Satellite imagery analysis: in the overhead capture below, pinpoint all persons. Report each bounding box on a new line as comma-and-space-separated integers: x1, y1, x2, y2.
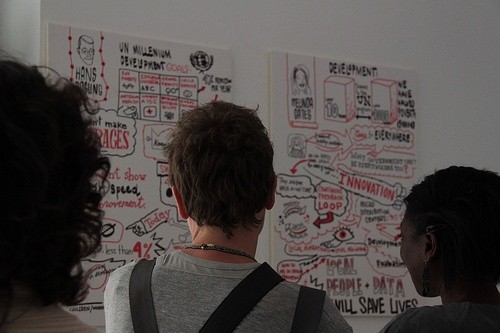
0, 47, 112, 333
375, 165, 500, 333
102, 96, 356, 333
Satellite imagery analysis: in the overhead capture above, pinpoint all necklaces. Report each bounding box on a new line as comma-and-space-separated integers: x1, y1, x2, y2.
183, 243, 258, 264
